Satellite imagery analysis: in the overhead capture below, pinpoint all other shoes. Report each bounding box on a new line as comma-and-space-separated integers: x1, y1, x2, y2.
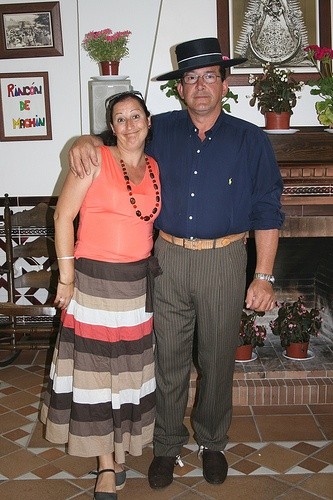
114, 463, 127, 490
202, 446, 229, 484
148, 454, 177, 489
88, 469, 118, 500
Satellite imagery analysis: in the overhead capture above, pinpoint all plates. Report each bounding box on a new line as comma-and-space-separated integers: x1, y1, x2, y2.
90, 76, 130, 81
263, 128, 301, 134
282, 349, 315, 360
235, 351, 258, 363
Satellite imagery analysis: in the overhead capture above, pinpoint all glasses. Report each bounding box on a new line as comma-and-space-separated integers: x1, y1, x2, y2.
105, 90, 146, 113
182, 73, 222, 84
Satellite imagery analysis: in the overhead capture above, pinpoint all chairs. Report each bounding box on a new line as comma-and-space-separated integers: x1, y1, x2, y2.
0, 194, 79, 368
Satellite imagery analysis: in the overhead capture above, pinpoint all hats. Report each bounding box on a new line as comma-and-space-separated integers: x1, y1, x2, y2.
155, 37, 249, 81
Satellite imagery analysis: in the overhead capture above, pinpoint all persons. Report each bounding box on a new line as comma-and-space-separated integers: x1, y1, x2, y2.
38, 91, 161, 500
68, 38, 284, 489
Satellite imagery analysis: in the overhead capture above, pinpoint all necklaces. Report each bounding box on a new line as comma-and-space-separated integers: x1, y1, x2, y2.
118, 146, 160, 222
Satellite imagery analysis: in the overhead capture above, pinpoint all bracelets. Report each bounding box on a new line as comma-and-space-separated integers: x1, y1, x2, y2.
255, 272, 275, 286
57, 256, 76, 260
58, 279, 75, 286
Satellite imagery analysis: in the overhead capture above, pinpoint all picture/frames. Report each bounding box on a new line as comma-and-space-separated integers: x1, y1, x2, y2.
0, 2, 63, 60
215, 0, 333, 87
0, 72, 52, 142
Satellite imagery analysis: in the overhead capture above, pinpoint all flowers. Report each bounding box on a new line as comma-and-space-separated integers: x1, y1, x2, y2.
246, 63, 304, 114
270, 296, 324, 347
302, 45, 333, 128
82, 29, 131, 62
238, 311, 268, 348
223, 56, 230, 61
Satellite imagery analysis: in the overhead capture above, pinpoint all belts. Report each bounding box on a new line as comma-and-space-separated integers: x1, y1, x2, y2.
158, 229, 250, 251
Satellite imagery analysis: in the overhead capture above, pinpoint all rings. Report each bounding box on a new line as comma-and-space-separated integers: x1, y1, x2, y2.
60, 298, 64, 302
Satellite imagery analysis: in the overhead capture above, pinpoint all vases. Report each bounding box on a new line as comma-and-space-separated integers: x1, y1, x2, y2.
98, 61, 121, 76
263, 111, 292, 130
236, 336, 253, 361
286, 335, 311, 359
